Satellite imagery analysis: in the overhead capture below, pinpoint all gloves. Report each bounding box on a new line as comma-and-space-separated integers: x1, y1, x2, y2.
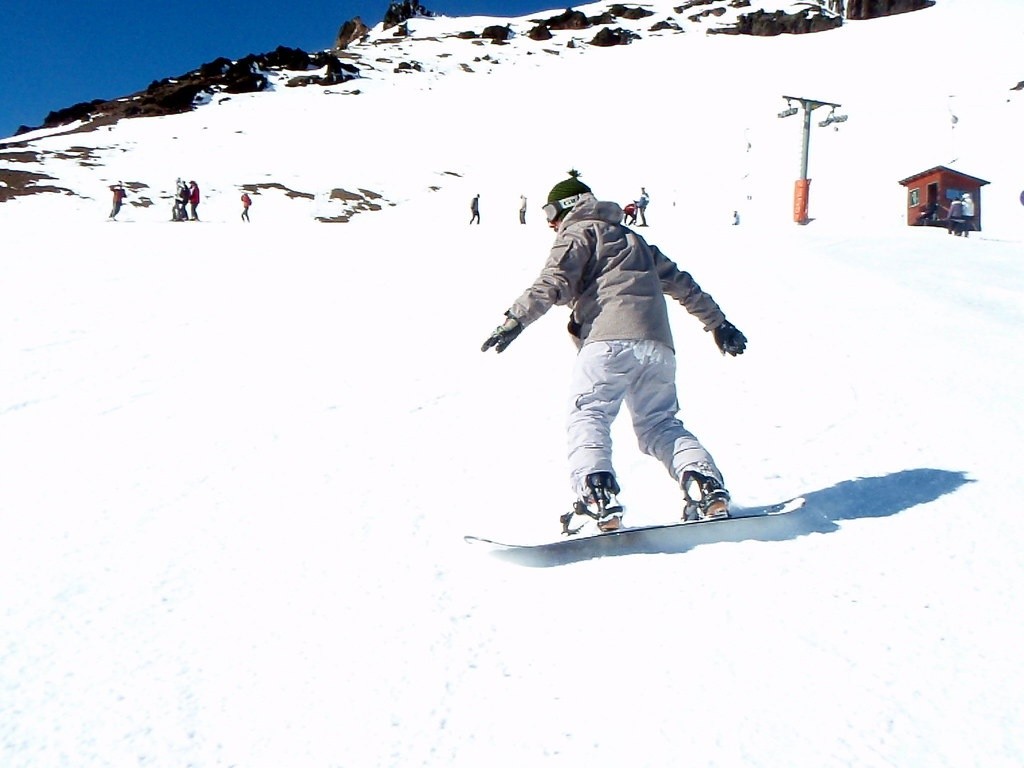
710, 319, 748, 357
481, 314, 523, 354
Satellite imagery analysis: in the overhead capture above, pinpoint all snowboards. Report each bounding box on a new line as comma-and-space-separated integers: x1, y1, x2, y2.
461, 495, 808, 551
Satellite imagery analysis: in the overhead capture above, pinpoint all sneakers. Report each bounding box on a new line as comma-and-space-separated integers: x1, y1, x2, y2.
560, 494, 625, 535
682, 477, 729, 521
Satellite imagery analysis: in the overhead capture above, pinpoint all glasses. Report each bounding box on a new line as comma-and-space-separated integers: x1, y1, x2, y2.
542, 192, 594, 222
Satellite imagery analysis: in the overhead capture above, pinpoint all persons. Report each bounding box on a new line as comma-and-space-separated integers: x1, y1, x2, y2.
947, 196, 963, 236
732, 211, 740, 225
180, 181, 191, 221
187, 181, 200, 221
634, 187, 649, 226
109, 180, 126, 221
623, 204, 639, 225
480, 178, 748, 536
519, 195, 528, 224
469, 194, 480, 225
958, 193, 974, 236
241, 193, 252, 222
171, 179, 183, 222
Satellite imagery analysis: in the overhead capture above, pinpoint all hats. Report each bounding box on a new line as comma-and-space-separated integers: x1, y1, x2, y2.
547, 169, 597, 222
